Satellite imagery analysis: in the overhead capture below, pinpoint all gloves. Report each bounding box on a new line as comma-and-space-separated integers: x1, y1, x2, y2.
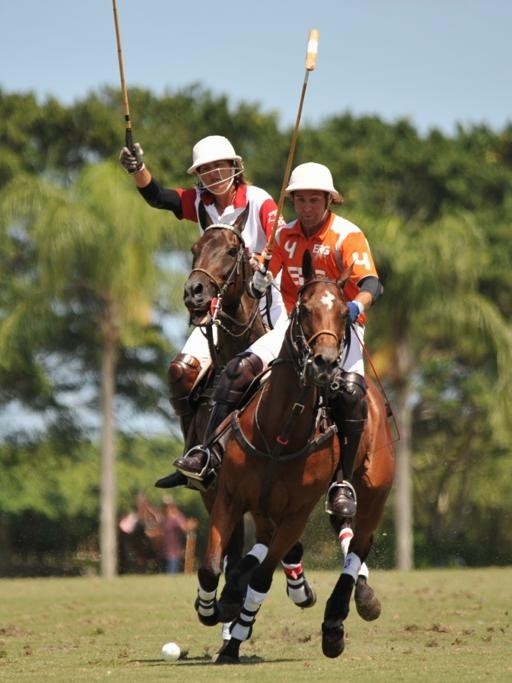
347, 302, 359, 323
253, 270, 273, 293
119, 143, 145, 174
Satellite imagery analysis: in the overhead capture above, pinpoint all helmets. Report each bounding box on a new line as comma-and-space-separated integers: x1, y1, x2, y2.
285, 162, 338, 199
186, 135, 241, 174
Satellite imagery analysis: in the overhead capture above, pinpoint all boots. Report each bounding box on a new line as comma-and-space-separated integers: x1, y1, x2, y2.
330, 480, 357, 518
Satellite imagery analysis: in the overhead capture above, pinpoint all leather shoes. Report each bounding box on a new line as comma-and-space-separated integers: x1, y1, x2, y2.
155, 452, 218, 491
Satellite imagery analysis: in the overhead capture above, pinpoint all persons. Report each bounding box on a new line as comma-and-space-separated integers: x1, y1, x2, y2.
116, 134, 288, 488
173, 162, 388, 518
156, 493, 198, 574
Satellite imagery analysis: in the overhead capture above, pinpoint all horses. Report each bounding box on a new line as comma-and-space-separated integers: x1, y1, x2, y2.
181, 197, 318, 665
193, 247, 400, 667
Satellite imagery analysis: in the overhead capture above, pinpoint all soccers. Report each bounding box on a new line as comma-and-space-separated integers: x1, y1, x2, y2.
162, 643, 181, 663
